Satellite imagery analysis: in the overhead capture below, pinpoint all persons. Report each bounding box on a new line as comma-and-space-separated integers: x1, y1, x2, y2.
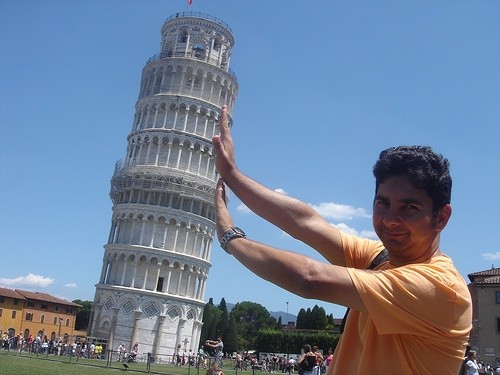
214, 105, 473, 375
0, 325, 333, 375
459, 342, 500, 375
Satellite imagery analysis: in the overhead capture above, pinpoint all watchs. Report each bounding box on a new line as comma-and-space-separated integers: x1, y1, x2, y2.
219, 226, 247, 256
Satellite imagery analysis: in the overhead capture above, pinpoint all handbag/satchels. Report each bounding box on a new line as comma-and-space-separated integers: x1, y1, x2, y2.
300, 355, 313, 372
